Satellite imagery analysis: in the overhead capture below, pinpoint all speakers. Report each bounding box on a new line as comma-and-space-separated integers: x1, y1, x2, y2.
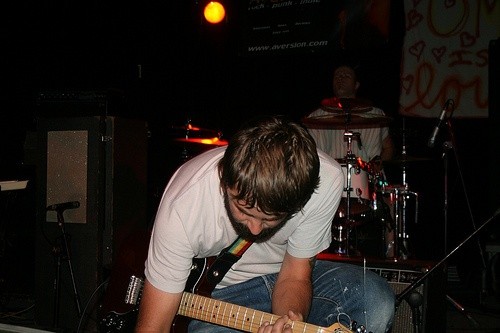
363, 263, 447, 333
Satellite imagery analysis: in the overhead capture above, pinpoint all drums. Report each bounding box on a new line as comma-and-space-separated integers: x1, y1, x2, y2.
334, 158, 422, 263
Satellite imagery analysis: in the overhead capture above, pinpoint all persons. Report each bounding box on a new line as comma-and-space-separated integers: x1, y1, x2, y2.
133, 119, 396, 333
303, 61, 396, 259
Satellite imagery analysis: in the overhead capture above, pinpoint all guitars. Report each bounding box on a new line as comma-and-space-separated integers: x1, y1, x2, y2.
75, 256, 373, 333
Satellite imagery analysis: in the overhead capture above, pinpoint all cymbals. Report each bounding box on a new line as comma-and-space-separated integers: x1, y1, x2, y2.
320, 95, 375, 114
301, 114, 393, 131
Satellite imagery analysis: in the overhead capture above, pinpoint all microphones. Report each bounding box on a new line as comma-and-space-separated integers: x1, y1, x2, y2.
431, 99, 449, 143
48, 201, 80, 211
